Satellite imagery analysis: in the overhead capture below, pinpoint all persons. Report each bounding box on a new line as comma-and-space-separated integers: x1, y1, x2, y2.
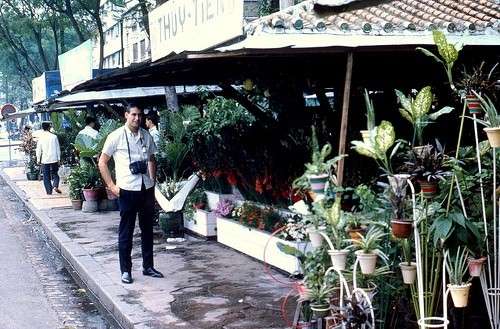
145, 114, 160, 151
75, 116, 98, 163
98, 102, 163, 284
36, 122, 64, 194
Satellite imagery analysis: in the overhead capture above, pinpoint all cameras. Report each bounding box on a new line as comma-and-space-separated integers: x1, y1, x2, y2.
129, 160, 147, 175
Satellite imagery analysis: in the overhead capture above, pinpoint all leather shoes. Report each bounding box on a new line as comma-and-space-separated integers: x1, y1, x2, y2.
143, 267, 163, 277
121, 272, 132, 283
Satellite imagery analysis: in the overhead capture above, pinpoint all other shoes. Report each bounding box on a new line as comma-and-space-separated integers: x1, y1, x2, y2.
54, 187, 62, 193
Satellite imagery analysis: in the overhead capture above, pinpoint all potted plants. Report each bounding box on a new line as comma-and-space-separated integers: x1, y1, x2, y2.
26, 30, 500, 329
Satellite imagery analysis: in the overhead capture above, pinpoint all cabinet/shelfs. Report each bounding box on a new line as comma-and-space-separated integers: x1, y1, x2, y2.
215, 200, 324, 276
182, 190, 231, 236
